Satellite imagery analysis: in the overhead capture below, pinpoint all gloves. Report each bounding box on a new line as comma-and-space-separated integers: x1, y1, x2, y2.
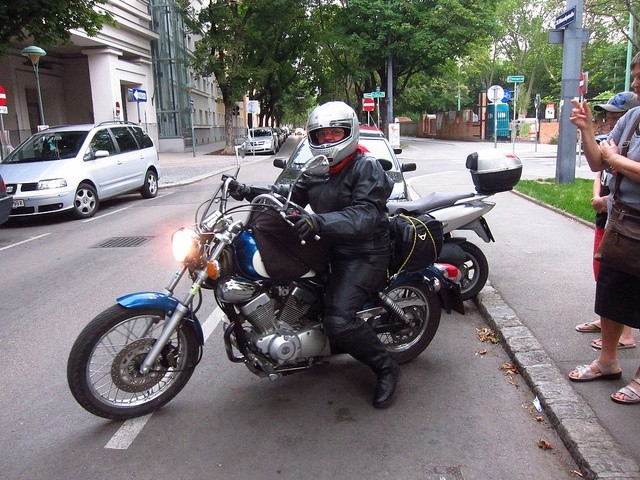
228, 184, 251, 201
285, 206, 321, 237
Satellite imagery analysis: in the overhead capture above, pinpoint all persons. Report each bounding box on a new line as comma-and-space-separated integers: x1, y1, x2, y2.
508, 119, 513, 141
228, 101, 400, 409
569, 54, 640, 405
514, 120, 521, 141
575, 91, 639, 350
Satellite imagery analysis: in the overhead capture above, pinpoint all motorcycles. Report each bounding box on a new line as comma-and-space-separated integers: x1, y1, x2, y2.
67, 136, 467, 422
380, 150, 523, 307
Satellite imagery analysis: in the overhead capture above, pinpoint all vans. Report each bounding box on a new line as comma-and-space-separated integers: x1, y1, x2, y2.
3, 121, 162, 222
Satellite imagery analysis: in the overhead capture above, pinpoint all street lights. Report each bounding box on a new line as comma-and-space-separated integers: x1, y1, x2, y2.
20, 45, 48, 126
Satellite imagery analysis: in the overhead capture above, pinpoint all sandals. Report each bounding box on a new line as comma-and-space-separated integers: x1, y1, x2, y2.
610, 377, 640, 404
568, 359, 623, 382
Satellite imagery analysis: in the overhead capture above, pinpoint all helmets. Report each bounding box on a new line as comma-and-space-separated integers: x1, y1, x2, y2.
306, 101, 360, 167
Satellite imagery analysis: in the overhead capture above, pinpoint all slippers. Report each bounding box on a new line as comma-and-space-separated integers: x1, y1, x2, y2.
575, 322, 601, 332
591, 339, 637, 350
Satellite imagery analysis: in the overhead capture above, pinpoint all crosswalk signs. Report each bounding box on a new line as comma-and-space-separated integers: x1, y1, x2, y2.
501, 89, 512, 103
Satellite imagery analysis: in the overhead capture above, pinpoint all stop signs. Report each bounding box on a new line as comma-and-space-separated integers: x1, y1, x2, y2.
113, 101, 120, 116
578, 73, 589, 100
362, 98, 375, 112
0, 85, 8, 115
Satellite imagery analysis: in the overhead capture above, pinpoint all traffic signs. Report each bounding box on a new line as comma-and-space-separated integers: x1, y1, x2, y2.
128, 88, 147, 102
363, 91, 385, 98
247, 100, 259, 114
507, 76, 525, 83
534, 95, 542, 111
555, 5, 576, 30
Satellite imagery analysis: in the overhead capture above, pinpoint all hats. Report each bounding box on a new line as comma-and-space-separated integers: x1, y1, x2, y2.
593, 91, 639, 113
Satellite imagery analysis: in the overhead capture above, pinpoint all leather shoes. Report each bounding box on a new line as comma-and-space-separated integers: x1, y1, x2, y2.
371, 359, 400, 408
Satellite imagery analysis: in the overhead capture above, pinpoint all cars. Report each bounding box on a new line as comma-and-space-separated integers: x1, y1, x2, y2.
274, 126, 293, 143
244, 127, 279, 155
295, 128, 307, 136
271, 135, 418, 203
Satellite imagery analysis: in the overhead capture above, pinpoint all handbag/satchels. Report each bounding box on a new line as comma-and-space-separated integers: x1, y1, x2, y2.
389, 208, 443, 272
594, 114, 640, 279
